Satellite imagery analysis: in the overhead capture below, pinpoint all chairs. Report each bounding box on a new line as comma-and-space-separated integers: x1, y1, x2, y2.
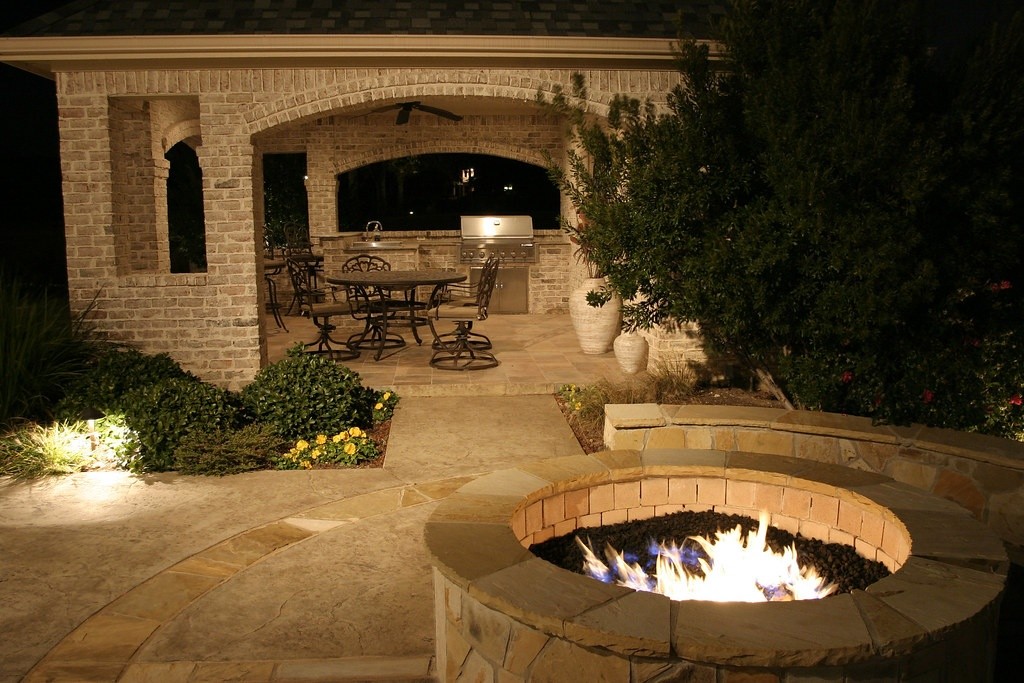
255, 221, 500, 371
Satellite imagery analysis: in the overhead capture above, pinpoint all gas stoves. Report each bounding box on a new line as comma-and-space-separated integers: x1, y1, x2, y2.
458, 216, 534, 263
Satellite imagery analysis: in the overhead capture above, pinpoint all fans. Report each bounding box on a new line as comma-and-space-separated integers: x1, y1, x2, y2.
372, 100, 462, 126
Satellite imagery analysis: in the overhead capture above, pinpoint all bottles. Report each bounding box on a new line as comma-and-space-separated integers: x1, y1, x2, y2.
373, 224, 380, 241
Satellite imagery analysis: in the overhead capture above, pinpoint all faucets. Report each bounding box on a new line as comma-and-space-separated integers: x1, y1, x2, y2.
366, 221, 382, 241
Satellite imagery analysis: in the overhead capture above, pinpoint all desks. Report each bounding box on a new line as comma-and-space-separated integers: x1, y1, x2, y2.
324, 271, 467, 363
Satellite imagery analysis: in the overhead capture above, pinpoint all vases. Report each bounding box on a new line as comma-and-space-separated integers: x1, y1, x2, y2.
614, 327, 648, 376
568, 277, 624, 354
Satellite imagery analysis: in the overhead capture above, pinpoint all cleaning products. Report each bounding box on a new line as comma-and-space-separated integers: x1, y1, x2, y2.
372, 224, 381, 241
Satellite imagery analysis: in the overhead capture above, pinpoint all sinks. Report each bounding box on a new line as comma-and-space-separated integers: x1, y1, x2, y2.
352, 242, 403, 247
